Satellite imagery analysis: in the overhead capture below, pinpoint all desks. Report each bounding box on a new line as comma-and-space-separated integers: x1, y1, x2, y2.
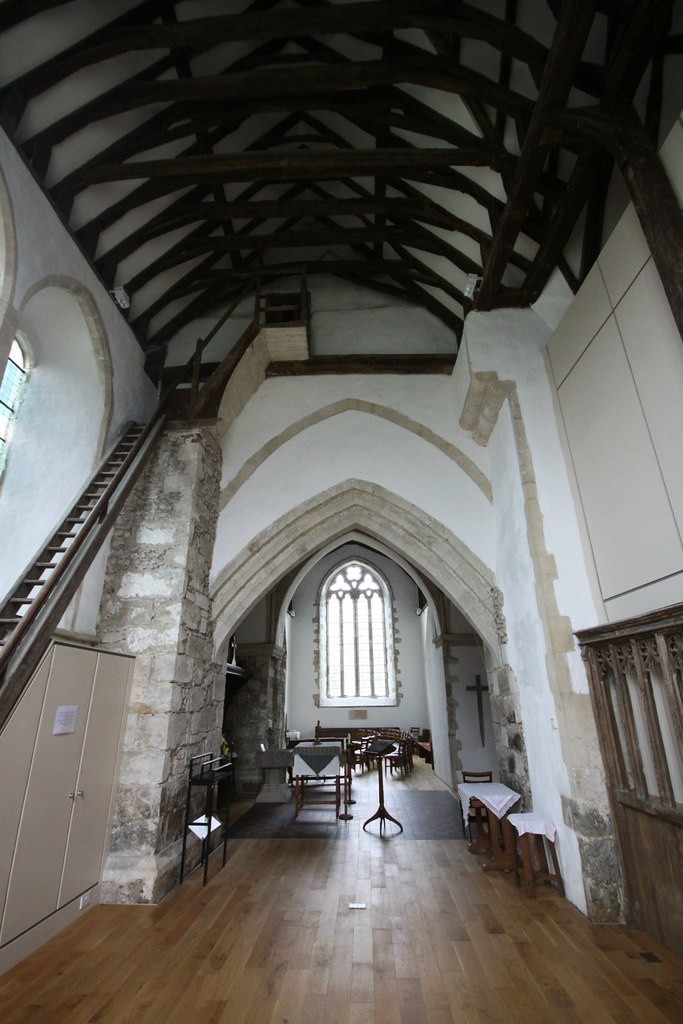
458, 783, 521, 873
294, 741, 340, 819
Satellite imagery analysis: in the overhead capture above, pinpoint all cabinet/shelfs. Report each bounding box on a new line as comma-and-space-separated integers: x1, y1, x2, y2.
0, 638, 137, 977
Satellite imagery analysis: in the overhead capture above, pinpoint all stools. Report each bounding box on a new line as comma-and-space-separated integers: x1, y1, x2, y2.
507, 814, 564, 899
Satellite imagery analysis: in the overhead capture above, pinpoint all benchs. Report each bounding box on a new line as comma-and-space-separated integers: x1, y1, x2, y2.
418, 727, 434, 770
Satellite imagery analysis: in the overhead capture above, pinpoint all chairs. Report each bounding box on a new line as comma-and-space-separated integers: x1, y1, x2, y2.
459, 771, 493, 848
346, 727, 419, 776
